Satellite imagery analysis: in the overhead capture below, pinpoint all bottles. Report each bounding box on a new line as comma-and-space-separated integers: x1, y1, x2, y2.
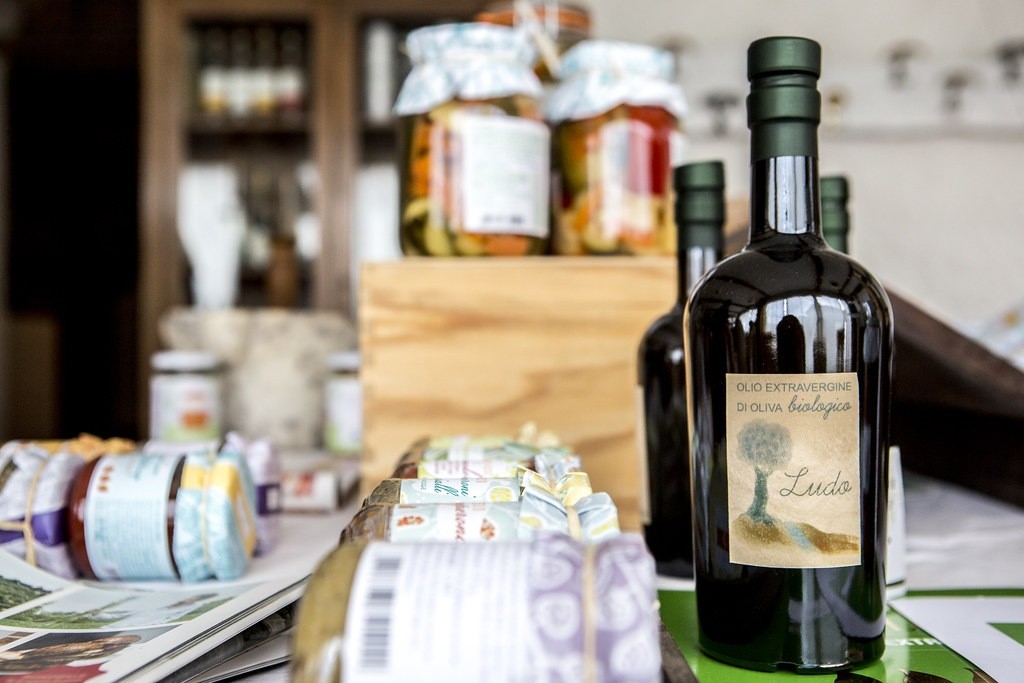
636, 161, 730, 575
0, 349, 364, 582
290, 420, 664, 683
816, 177, 906, 587
400, 1, 683, 257
682, 37, 894, 675
199, 26, 306, 117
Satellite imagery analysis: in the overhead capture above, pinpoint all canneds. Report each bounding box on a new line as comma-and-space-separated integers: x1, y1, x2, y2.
321, 351, 363, 453
145, 351, 225, 451
0, 431, 280, 583
289, 426, 663, 683
390, 0, 684, 256
